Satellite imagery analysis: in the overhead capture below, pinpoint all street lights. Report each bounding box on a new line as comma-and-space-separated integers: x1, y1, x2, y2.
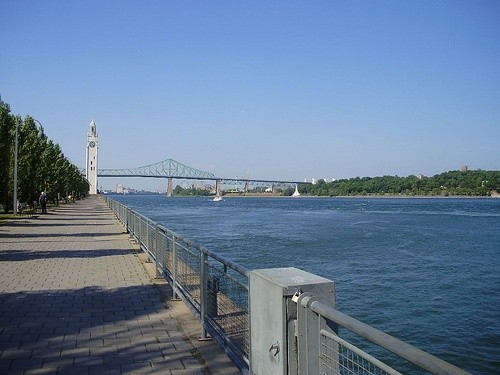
13, 119, 44, 216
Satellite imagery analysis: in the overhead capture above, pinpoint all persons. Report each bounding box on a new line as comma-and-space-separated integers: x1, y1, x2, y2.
39, 192, 47, 214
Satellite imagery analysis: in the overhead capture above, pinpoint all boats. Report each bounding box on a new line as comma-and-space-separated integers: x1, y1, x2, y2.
213, 196, 223, 201
